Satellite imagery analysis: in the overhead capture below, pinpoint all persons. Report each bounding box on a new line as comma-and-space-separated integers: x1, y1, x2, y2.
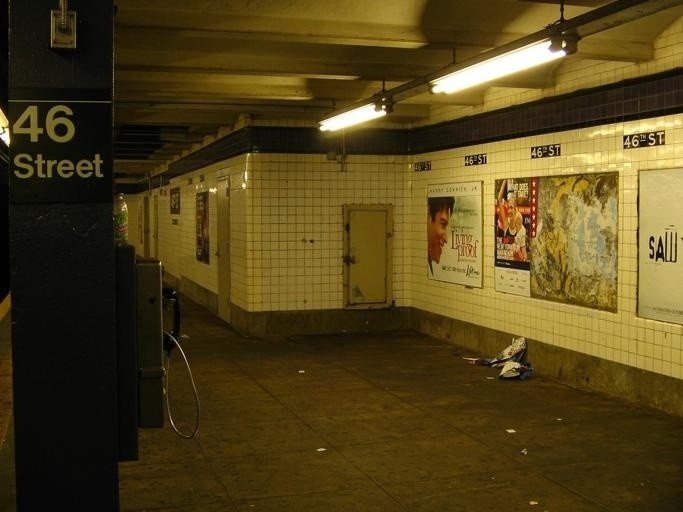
496, 180, 527, 263
427, 197, 455, 278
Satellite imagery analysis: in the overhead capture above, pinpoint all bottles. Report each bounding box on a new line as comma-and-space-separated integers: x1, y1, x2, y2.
113, 193, 129, 249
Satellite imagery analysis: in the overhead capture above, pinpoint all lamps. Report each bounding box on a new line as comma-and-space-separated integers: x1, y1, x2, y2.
0, 108, 11, 150
317, 94, 393, 133
428, 27, 577, 95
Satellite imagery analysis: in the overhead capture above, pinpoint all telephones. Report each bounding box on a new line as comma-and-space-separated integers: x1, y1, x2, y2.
162, 287, 181, 352
